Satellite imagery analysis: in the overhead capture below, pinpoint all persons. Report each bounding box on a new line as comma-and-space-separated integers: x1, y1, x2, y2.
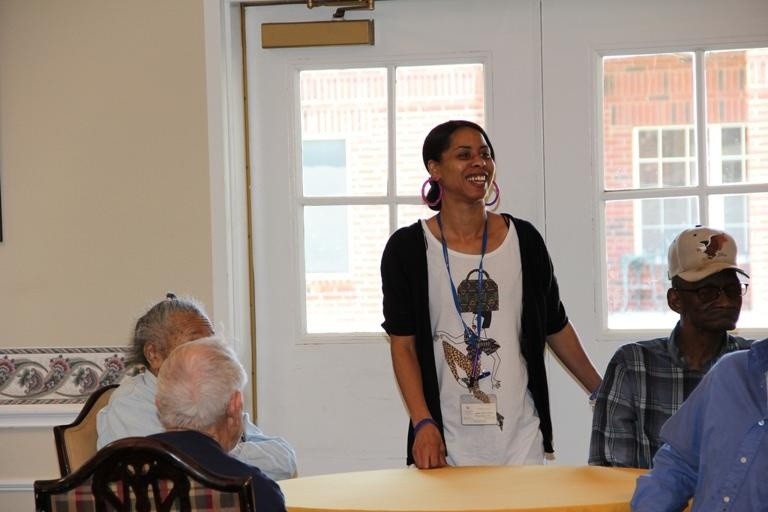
380, 120, 606, 470
97, 293, 298, 482
628, 339, 766, 512
140, 335, 287, 511
588, 223, 759, 470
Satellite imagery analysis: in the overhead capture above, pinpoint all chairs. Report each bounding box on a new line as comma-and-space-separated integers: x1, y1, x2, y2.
51, 380, 126, 482
29, 434, 262, 511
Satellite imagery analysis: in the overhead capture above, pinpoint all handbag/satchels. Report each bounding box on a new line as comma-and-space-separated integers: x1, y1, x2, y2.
458, 268, 498, 311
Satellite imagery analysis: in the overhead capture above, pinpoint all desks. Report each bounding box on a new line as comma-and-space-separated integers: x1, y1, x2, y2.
274, 462, 701, 510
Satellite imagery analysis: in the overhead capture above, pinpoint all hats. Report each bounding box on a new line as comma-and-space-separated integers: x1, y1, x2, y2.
668, 227, 749, 282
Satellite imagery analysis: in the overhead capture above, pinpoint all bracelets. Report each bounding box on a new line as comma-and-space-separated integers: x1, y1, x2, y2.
413, 418, 439, 435
589, 384, 599, 402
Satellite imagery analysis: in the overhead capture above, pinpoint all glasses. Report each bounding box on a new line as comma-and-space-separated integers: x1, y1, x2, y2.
676, 282, 747, 303
